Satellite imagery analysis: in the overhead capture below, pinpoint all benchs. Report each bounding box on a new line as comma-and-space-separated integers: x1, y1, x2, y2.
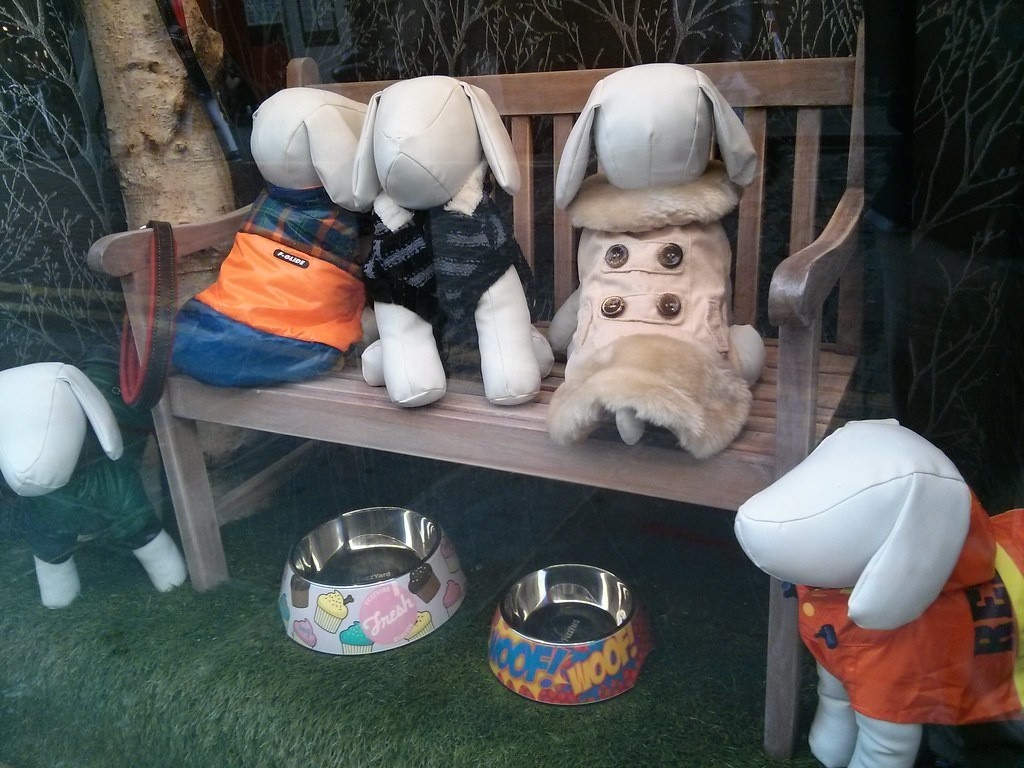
86, 17, 899, 760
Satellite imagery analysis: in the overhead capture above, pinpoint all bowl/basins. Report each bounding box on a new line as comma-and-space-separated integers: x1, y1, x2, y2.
488, 564, 658, 706
277, 506, 468, 654
